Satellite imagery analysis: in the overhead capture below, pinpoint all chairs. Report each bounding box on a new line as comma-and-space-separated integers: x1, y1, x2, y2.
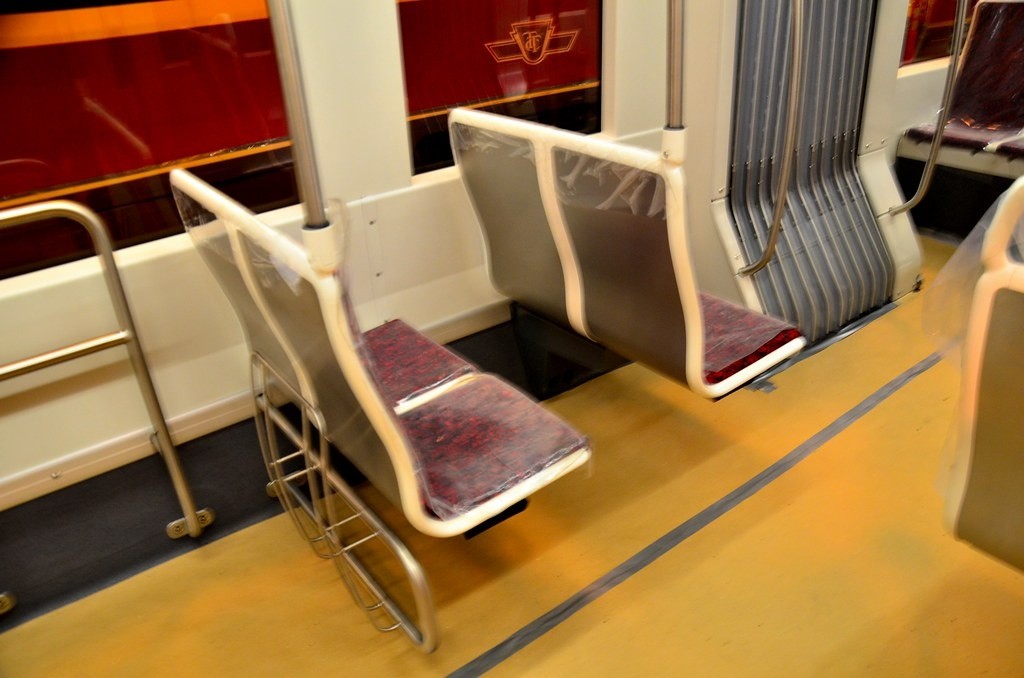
170, 0, 1024, 574
